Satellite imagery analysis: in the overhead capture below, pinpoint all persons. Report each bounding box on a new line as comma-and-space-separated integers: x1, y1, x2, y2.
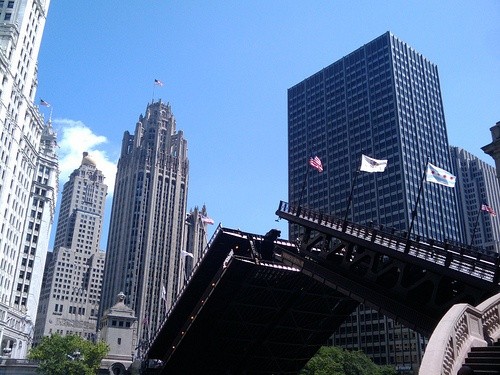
457, 364, 474, 375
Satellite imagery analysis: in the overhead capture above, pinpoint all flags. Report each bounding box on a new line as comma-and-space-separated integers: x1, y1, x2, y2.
40, 98, 51, 108
308, 153, 323, 173
154, 79, 163, 86
360, 154, 388, 172
201, 214, 213, 225
425, 161, 456, 187
481, 200, 497, 217
